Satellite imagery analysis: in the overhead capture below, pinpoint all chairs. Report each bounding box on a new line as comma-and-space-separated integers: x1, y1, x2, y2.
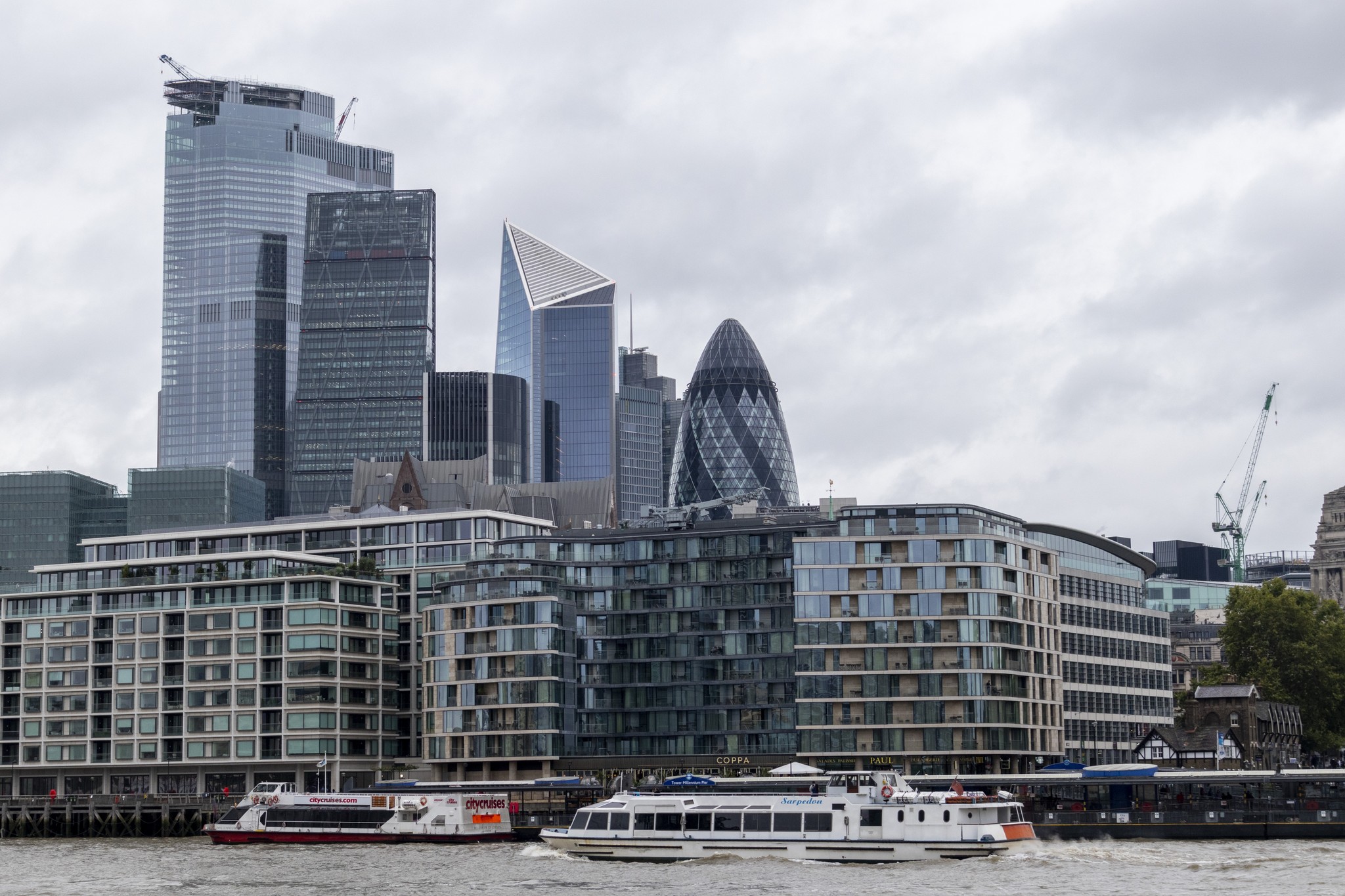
849, 690, 855, 698
903, 636, 913, 643
846, 664, 851, 671
875, 583, 881, 590
973, 743, 978, 750
958, 582, 968, 588
943, 662, 949, 669
895, 662, 900, 670
861, 744, 867, 752
839, 664, 844, 671
957, 716, 962, 723
862, 583, 868, 590
879, 557, 884, 563
950, 608, 968, 615
871, 743, 876, 751
857, 663, 862, 671
902, 663, 907, 670
856, 717, 860, 725
948, 635, 953, 642
898, 609, 911, 616
957, 661, 963, 669
875, 557, 879, 564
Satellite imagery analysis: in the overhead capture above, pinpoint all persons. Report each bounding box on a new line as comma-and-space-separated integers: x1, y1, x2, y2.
1208, 788, 1213, 799
652, 787, 661, 796
1200, 787, 1205, 799
1246, 791, 1254, 810
809, 781, 819, 797
1221, 792, 1233, 809
737, 772, 741, 777
332, 789, 336, 794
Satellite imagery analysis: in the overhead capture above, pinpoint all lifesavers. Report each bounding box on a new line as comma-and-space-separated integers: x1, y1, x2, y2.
420, 796, 427, 806
268, 798, 273, 806
273, 796, 278, 804
254, 796, 259, 805
881, 786, 892, 798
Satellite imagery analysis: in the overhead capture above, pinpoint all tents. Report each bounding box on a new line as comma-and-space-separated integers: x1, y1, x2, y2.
768, 761, 825, 778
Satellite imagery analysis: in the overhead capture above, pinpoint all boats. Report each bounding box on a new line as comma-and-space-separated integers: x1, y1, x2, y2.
538, 760, 1041, 864
200, 781, 517, 844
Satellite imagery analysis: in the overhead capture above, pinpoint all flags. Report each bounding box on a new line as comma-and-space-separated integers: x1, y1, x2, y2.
1218, 732, 1226, 760
951, 780, 964, 796
317, 754, 327, 767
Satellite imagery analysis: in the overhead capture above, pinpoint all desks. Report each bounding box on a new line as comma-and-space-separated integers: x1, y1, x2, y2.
870, 587, 874, 590
851, 664, 856, 671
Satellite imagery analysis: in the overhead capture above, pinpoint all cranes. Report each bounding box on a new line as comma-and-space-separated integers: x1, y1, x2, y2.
1212, 381, 1280, 583
